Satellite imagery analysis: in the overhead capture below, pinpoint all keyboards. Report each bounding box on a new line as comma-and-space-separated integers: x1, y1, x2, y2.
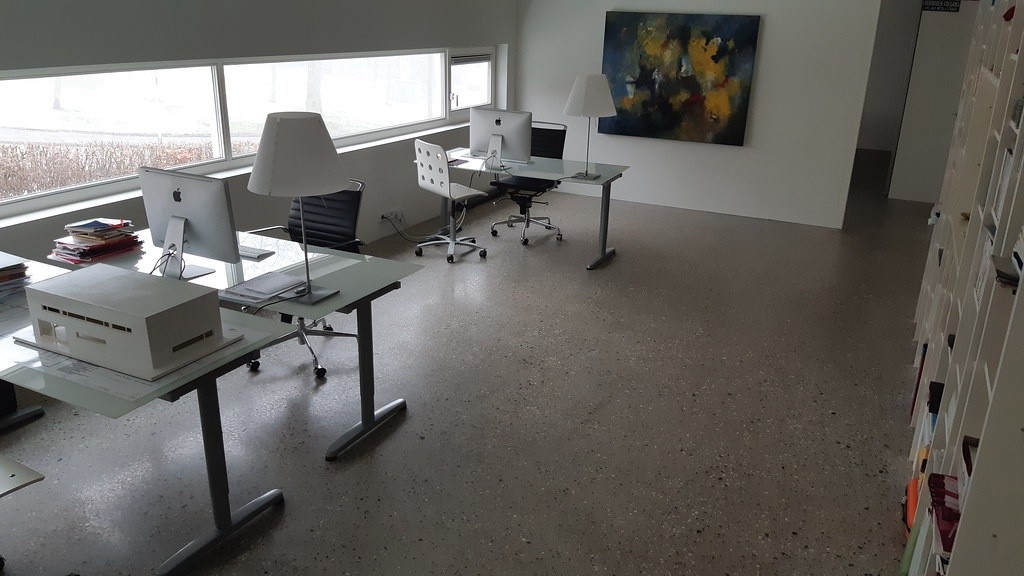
239, 245, 275, 259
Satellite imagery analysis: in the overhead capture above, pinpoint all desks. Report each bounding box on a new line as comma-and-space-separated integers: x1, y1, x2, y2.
414, 147, 630, 270
0, 250, 299, 576
81, 228, 425, 470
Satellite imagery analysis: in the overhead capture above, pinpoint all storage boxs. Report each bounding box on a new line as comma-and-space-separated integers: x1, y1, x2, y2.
24, 262, 224, 370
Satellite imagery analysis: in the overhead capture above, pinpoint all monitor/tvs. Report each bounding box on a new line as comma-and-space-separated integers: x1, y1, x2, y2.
137, 166, 240, 281
470, 107, 532, 169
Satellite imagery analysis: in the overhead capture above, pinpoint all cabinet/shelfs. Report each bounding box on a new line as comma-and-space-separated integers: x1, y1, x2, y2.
898, 0, 1024, 576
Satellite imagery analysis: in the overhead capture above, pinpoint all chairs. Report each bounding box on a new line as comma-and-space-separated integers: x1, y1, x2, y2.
415, 139, 486, 261
491, 119, 568, 245
242, 179, 366, 377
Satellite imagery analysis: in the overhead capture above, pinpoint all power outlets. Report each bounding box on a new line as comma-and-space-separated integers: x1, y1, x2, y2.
377, 206, 405, 223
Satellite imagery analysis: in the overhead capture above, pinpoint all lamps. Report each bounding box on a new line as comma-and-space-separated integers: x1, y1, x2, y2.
246, 112, 353, 306
563, 73, 617, 182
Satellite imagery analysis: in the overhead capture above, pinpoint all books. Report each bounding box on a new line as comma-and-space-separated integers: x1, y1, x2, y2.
923, 507, 962, 576
0, 252, 32, 298
52, 218, 142, 264
